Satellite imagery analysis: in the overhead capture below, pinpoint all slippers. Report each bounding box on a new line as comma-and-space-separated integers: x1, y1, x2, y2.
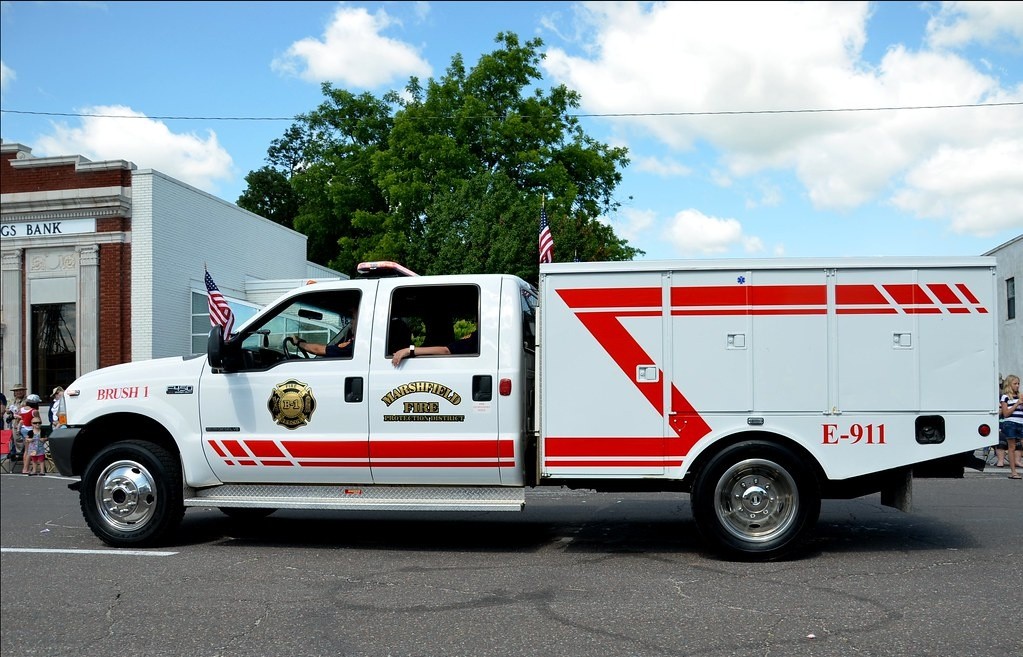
993, 459, 1004, 468
1008, 475, 1022, 479
1015, 460, 1023, 468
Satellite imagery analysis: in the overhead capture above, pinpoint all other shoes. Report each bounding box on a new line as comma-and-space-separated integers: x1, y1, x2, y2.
39, 471, 45, 475
22, 470, 31, 474
28, 471, 38, 476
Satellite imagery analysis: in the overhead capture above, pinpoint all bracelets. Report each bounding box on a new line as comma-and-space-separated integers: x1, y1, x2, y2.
297, 339, 306, 346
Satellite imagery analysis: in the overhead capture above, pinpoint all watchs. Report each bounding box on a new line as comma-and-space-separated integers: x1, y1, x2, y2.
409, 345, 414, 357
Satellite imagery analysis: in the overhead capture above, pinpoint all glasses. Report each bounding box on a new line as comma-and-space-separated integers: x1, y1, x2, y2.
31, 421, 42, 425
471, 320, 477, 324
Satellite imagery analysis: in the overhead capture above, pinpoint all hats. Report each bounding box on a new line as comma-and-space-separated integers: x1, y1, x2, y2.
50, 386, 65, 398
999, 373, 1002, 383
10, 384, 27, 390
27, 394, 42, 403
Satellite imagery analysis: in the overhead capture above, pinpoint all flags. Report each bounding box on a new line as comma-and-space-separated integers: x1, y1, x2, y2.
539, 209, 554, 263
205, 272, 234, 340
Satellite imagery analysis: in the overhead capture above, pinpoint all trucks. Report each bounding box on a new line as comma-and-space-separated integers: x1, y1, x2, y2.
48, 258, 1003, 566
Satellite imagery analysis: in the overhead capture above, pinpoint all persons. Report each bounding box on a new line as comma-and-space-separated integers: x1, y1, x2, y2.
291, 305, 357, 358
991, 373, 1023, 479
0, 384, 64, 475
391, 310, 478, 365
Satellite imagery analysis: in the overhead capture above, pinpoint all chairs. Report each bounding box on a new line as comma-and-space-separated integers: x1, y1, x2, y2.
0, 430, 56, 474
